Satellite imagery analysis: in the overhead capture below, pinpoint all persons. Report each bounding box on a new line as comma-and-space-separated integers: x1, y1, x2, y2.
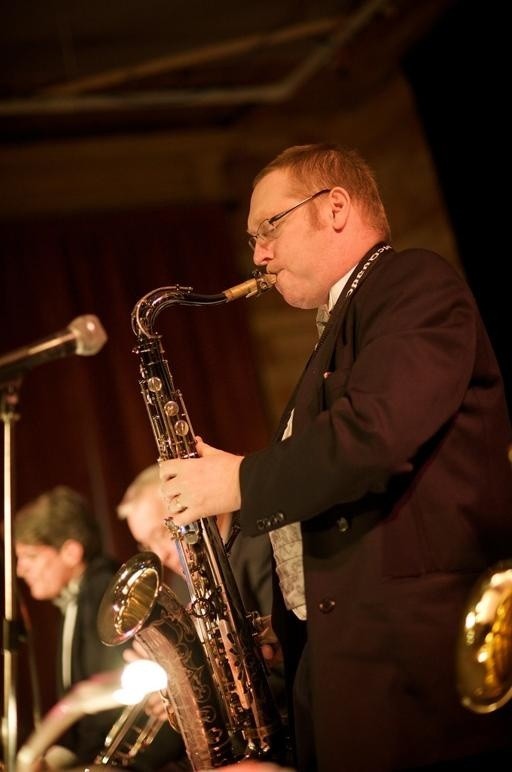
10, 484, 181, 772
155, 142, 512, 772
114, 453, 278, 771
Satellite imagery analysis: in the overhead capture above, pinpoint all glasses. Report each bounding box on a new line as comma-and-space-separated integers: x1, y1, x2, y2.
248, 187, 331, 253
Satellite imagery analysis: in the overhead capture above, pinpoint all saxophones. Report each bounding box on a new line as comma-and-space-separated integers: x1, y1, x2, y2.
98, 270, 296, 771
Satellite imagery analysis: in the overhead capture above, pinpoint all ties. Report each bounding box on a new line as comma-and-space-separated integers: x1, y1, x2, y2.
315, 304, 330, 340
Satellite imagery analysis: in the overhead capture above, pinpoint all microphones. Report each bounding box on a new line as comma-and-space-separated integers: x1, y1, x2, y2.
0, 314, 109, 377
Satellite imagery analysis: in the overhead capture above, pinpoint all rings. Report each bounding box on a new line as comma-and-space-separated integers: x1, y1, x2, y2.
173, 497, 185, 511
